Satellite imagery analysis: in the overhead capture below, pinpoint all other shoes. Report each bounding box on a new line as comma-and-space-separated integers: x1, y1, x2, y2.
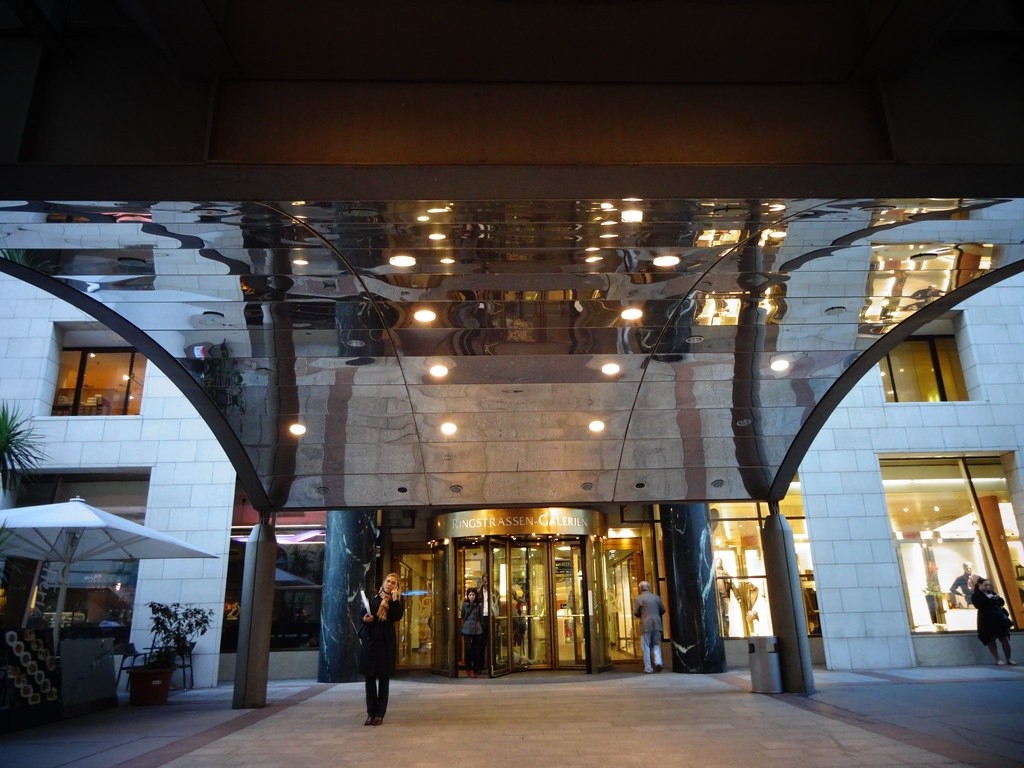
483, 664, 488, 670
465, 670, 472, 678
1006, 658, 1016, 665
995, 659, 1004, 665
654, 664, 662, 672
472, 671, 478, 678
493, 664, 498, 671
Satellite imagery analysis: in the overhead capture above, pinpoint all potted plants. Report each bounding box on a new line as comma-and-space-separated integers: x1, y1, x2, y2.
126, 601, 215, 706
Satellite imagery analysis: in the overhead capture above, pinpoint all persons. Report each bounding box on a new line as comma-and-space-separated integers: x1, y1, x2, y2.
950, 562, 982, 604
300, 609, 315, 623
970, 578, 1018, 666
911, 285, 946, 309
360, 573, 404, 726
464, 574, 501, 671
716, 558, 741, 637
461, 589, 483, 677
634, 581, 666, 673
27, 608, 48, 629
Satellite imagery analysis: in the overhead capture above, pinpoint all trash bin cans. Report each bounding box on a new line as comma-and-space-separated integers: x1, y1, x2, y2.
747, 636, 783, 694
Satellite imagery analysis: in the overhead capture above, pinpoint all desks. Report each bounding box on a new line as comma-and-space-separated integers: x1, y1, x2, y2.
49, 404, 74, 417
143, 645, 176, 668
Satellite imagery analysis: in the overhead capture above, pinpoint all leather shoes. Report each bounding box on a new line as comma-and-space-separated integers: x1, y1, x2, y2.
364, 714, 375, 725
372, 714, 382, 725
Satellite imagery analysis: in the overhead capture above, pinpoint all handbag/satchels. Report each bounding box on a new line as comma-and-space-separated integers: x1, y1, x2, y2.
357, 623, 369, 643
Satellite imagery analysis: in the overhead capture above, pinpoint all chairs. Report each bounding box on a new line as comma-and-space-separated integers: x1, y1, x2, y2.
173, 642, 197, 692
117, 642, 147, 692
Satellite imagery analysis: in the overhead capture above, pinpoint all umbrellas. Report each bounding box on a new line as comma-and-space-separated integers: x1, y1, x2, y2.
276, 569, 322, 589
0, 495, 220, 654
